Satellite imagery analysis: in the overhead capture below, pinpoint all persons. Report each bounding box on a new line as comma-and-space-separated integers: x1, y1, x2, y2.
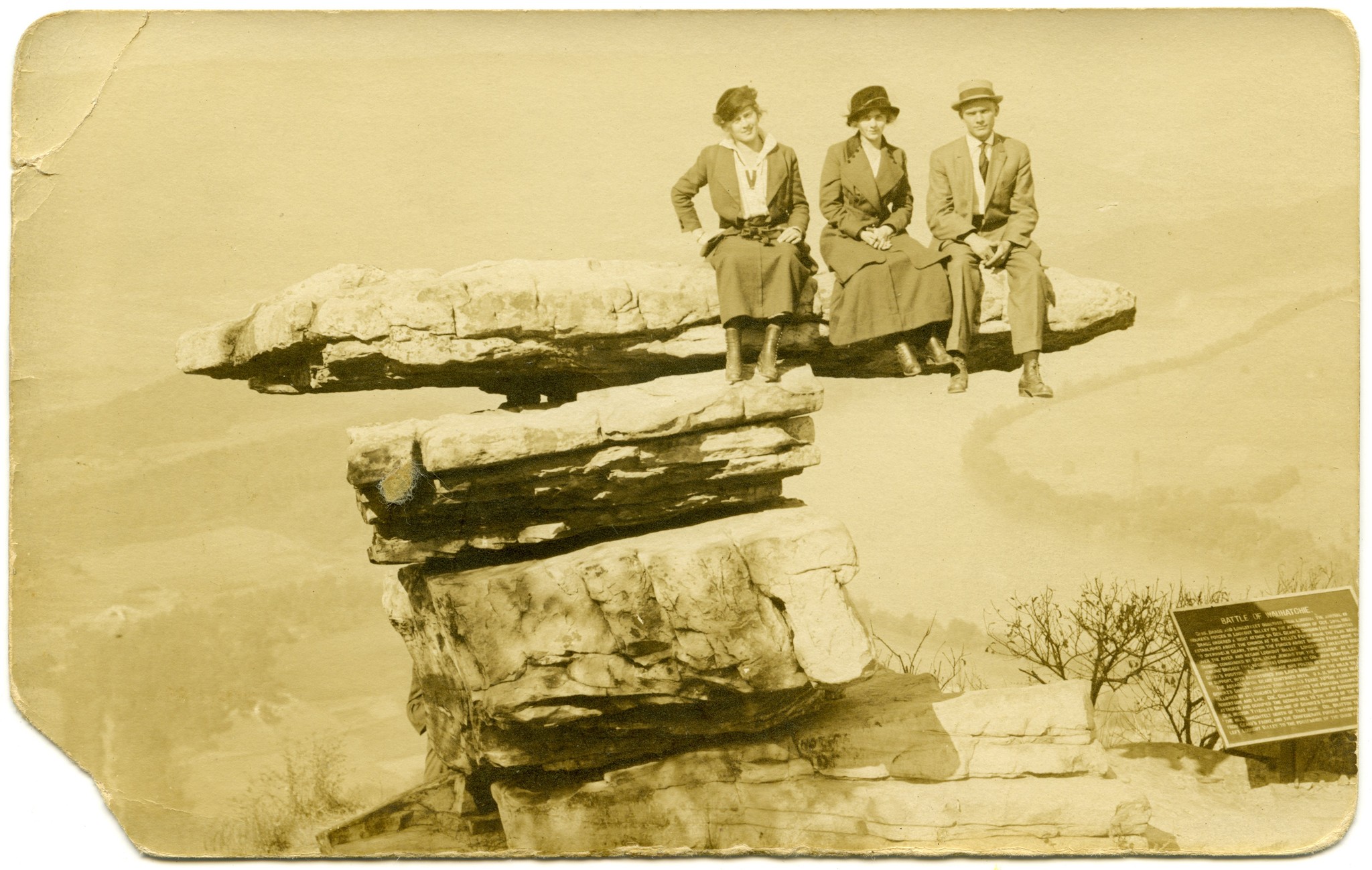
818, 86, 954, 376
925, 78, 1057, 398
669, 84, 818, 381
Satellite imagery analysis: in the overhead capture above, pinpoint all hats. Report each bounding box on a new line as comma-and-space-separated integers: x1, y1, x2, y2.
715, 85, 755, 121
846, 86, 899, 128
951, 79, 1003, 112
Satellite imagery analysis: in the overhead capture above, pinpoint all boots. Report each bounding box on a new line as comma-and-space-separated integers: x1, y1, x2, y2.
725, 329, 743, 381
757, 326, 783, 380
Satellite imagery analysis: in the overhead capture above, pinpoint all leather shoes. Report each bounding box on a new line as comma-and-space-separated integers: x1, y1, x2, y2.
1018, 370, 1053, 398
947, 358, 968, 393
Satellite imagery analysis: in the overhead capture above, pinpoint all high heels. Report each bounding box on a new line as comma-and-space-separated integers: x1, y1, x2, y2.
922, 322, 954, 366
886, 331, 922, 376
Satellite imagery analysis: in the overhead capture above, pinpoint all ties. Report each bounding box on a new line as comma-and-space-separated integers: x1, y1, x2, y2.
977, 142, 989, 185
